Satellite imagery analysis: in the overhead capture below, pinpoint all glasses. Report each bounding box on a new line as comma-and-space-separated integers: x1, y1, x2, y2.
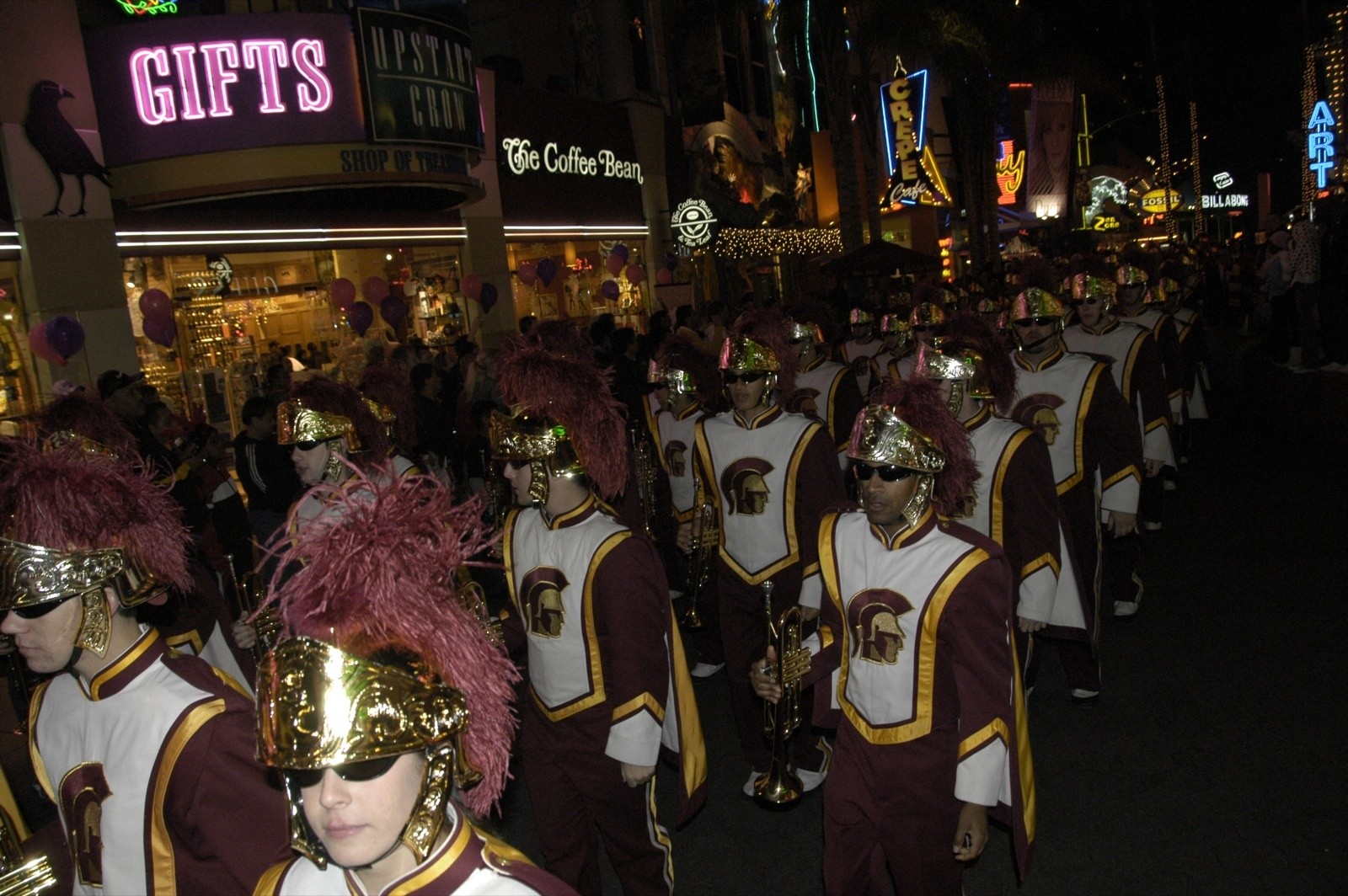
296, 434, 343, 451
853, 322, 868, 327
787, 338, 803, 345
1123, 283, 1143, 289
289, 755, 401, 787
651, 383, 667, 390
854, 464, 916, 481
10, 593, 80, 620
508, 459, 532, 470
1076, 298, 1100, 305
915, 325, 937, 332
1014, 317, 1057, 328
725, 370, 765, 385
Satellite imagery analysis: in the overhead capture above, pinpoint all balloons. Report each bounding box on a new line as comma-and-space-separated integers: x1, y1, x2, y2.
605, 254, 624, 277
536, 259, 556, 287
626, 265, 642, 287
612, 244, 628, 265
331, 278, 355, 309
663, 252, 678, 271
143, 309, 175, 348
460, 274, 481, 302
479, 283, 498, 314
139, 289, 173, 315
381, 295, 405, 330
519, 264, 537, 287
26, 316, 85, 367
363, 276, 388, 308
657, 269, 671, 284
602, 281, 619, 302
349, 302, 373, 337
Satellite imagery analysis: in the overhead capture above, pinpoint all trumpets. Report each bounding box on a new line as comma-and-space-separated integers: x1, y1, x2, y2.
679, 478, 720, 630
222, 551, 283, 671
479, 448, 507, 531
0, 804, 60, 896
754, 575, 815, 807
457, 577, 507, 650
629, 427, 661, 544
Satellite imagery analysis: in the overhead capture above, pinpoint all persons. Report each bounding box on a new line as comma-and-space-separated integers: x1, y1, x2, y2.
0, 455, 296, 896
1028, 101, 1072, 194
252, 452, 581, 896
101, 217, 1326, 896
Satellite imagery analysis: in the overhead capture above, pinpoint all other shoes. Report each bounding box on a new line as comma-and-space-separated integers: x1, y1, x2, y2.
1115, 600, 1139, 621
1071, 688, 1099, 705
795, 762, 828, 793
741, 770, 766, 800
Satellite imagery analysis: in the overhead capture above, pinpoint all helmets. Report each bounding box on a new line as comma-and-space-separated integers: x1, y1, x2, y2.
489, 348, 630, 507
275, 375, 396, 480
647, 234, 1221, 529
480, 334, 616, 482
250, 458, 521, 867
0, 393, 190, 656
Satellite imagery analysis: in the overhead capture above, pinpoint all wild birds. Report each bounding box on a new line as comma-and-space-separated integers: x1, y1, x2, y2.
22, 78, 116, 219
